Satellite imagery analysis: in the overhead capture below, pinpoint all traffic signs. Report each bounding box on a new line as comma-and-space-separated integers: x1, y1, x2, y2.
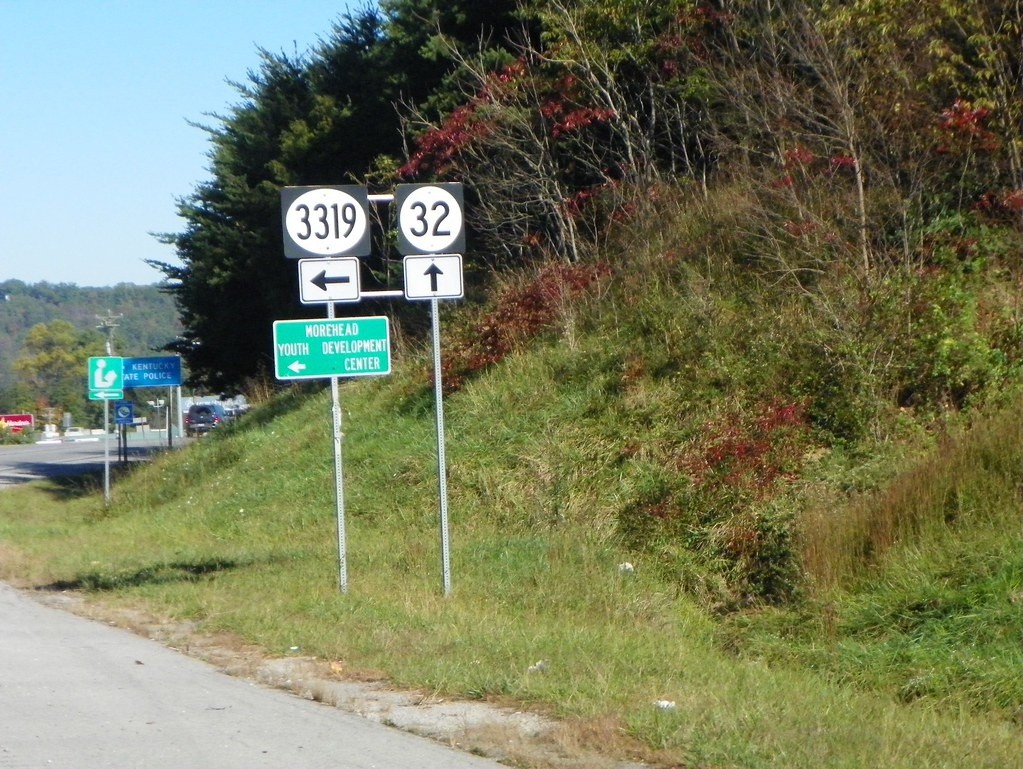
88, 357, 127, 402
272, 312, 391, 381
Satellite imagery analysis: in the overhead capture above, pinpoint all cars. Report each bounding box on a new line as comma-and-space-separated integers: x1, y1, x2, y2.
225, 409, 249, 425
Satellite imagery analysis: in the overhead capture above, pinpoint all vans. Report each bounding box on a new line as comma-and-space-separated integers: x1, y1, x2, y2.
185, 404, 227, 438
